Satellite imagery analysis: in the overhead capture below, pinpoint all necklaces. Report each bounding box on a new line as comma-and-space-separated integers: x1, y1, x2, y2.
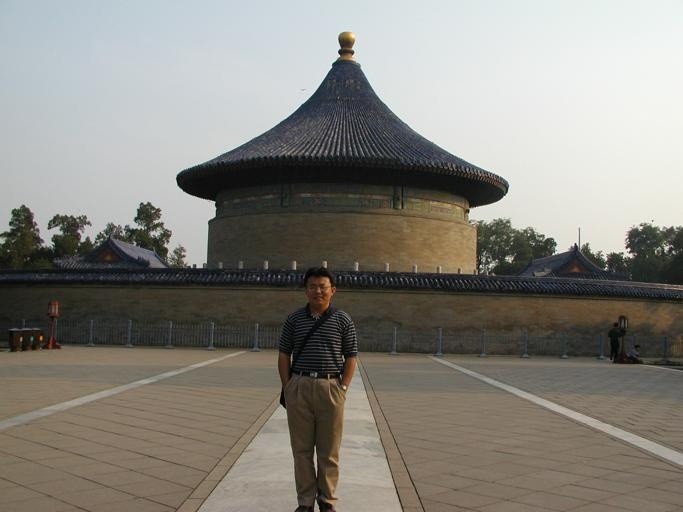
309, 306, 322, 321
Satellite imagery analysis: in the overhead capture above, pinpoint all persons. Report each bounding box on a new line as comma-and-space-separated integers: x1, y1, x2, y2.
626, 344, 643, 364
608, 322, 625, 363
278, 267, 358, 512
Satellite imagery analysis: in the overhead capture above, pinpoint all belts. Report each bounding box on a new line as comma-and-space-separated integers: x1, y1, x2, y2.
291, 369, 341, 379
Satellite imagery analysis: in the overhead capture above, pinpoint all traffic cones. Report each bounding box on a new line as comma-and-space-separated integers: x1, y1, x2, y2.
47, 335, 53, 350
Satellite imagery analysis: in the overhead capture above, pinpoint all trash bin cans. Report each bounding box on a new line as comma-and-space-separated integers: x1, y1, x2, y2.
8, 328, 44, 352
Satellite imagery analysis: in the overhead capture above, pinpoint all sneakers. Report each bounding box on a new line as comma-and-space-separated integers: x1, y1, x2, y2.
295, 506, 314, 512
319, 503, 336, 512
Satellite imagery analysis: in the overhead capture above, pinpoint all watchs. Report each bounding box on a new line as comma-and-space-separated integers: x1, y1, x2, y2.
340, 384, 346, 391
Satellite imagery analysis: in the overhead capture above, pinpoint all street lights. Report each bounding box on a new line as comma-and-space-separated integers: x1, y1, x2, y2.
613, 315, 629, 364
40, 300, 61, 349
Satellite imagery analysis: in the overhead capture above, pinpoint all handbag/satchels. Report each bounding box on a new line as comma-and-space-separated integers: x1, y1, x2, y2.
280, 389, 285, 408
